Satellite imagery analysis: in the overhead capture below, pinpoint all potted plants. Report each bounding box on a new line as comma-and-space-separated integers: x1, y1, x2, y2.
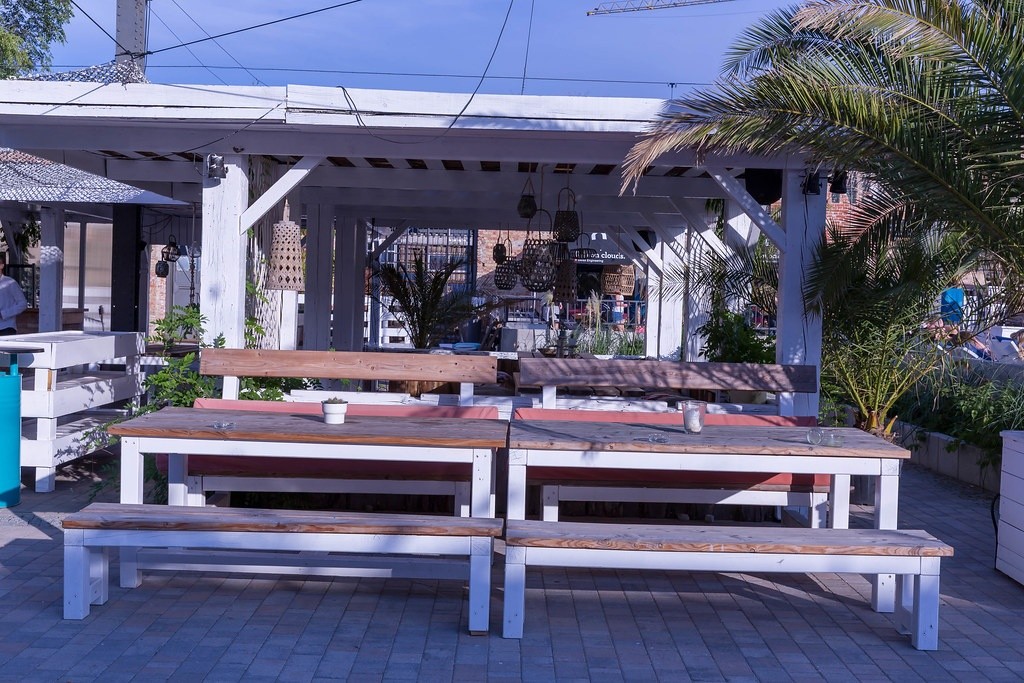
321, 397, 348, 425
820, 319, 972, 506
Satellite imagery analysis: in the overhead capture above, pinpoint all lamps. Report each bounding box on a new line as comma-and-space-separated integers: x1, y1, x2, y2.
193, 152, 229, 179
799, 167, 849, 195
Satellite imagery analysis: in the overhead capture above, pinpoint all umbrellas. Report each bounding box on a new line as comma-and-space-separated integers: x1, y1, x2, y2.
0, 146, 192, 231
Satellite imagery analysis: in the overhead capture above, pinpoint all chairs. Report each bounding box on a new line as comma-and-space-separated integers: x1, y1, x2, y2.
925, 336, 1024, 364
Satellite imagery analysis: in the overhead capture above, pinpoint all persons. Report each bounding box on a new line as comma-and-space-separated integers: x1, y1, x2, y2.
0, 259, 29, 336
919, 312, 1024, 363
614, 292, 628, 332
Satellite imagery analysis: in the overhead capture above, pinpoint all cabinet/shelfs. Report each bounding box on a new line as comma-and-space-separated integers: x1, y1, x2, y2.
0, 330, 146, 493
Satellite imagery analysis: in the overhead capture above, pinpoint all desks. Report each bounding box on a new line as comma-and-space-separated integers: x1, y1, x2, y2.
106, 405, 509, 586
506, 407, 911, 613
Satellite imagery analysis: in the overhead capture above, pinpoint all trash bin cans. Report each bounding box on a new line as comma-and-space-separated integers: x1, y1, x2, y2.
0, 346, 45, 509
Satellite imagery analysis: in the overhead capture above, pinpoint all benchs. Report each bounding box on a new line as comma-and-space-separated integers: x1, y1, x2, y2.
504, 358, 856, 529
61, 502, 505, 636
155, 347, 499, 517
502, 519, 955, 651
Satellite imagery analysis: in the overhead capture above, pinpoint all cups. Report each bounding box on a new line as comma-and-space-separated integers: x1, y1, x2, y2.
682, 400, 707, 434
806, 428, 824, 445
826, 434, 842, 446
